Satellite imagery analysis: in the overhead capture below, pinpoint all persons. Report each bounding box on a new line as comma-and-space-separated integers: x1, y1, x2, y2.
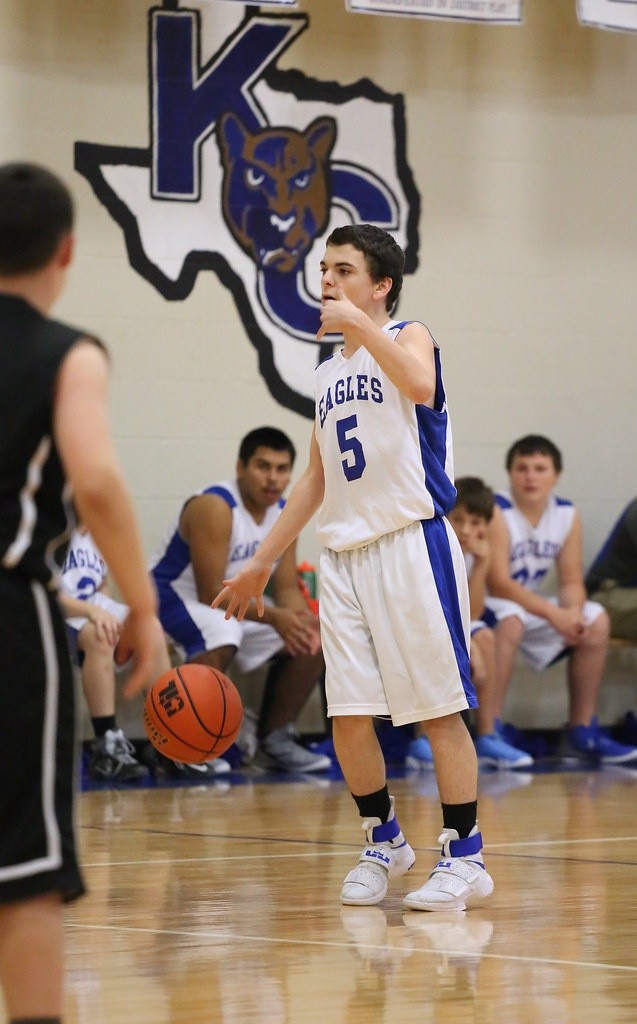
211, 224, 495, 914
405, 476, 533, 770
154, 427, 334, 772
586, 498, 636, 643
62, 493, 228, 779
1, 162, 171, 1023
483, 433, 636, 765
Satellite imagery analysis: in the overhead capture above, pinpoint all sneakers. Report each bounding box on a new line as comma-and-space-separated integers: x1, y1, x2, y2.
407, 735, 434, 770
206, 757, 232, 774
88, 725, 150, 783
471, 728, 535, 771
339, 794, 416, 905
143, 738, 217, 781
499, 722, 553, 762
403, 818, 495, 910
252, 728, 332, 775
558, 715, 637, 765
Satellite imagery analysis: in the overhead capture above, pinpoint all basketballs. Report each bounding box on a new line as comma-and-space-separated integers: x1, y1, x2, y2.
143, 662, 244, 765
